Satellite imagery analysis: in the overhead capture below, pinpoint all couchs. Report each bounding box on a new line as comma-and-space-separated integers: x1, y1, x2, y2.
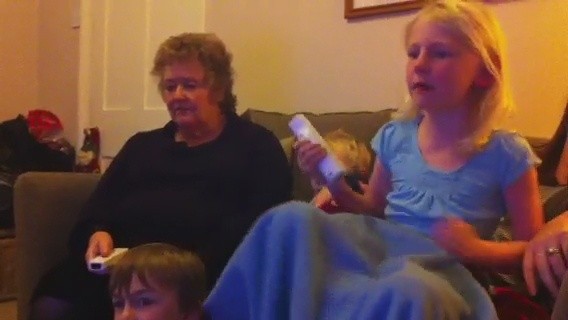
17, 110, 567, 319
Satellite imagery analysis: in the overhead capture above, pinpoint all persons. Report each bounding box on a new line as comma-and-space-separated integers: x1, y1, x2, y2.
25, 32, 292, 320
108, 243, 208, 320
522, 211, 568, 320
202, 0, 544, 320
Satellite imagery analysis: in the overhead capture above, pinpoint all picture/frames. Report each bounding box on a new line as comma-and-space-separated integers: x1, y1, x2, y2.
344, 1, 440, 21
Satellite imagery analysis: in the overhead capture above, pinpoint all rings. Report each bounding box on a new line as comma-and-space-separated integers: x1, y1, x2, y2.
545, 248, 561, 256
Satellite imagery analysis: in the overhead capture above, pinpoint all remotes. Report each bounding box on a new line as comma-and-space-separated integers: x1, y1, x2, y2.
88, 247, 129, 272
287, 114, 346, 183
